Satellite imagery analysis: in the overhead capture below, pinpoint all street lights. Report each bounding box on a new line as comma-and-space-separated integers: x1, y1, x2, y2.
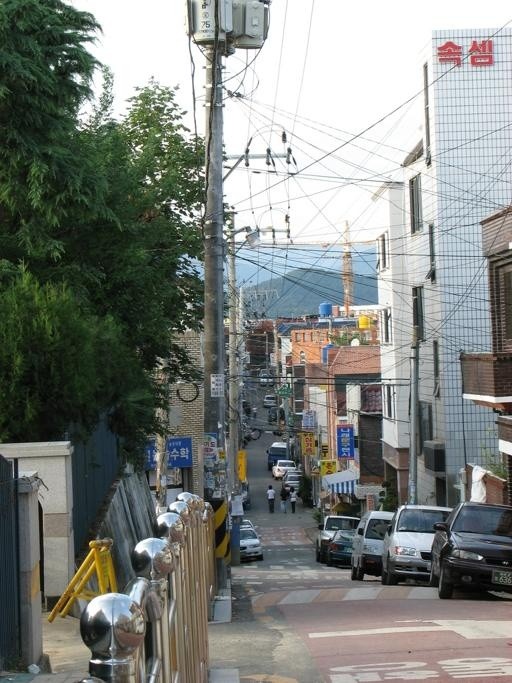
223, 225, 262, 252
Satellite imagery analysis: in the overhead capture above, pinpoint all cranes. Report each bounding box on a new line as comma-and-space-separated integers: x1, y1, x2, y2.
321, 220, 375, 317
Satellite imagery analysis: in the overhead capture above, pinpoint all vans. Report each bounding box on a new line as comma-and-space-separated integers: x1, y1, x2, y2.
351, 510, 395, 580
314, 515, 360, 562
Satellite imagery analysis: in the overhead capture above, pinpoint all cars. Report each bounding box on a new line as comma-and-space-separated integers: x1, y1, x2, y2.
381, 504, 454, 585
240, 519, 259, 529
259, 369, 303, 494
429, 500, 512, 599
242, 478, 251, 510
240, 528, 264, 561
327, 530, 356, 567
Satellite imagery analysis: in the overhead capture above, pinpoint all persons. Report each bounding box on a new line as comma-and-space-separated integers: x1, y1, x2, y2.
289, 486, 298, 514
251, 404, 259, 418
279, 483, 288, 513
266, 484, 276, 513
